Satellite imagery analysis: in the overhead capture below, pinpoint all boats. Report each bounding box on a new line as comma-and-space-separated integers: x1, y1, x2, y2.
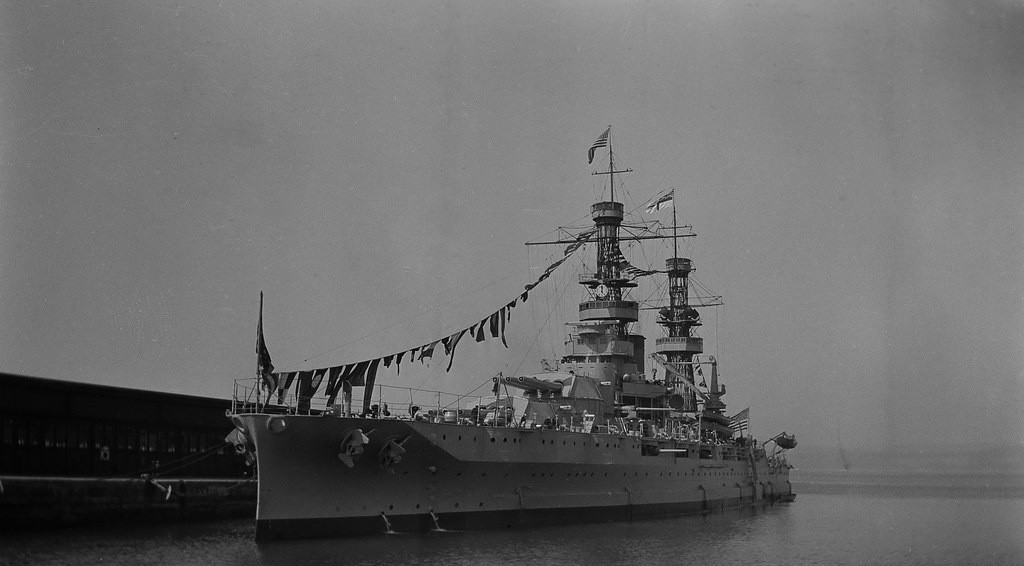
226, 124, 794, 546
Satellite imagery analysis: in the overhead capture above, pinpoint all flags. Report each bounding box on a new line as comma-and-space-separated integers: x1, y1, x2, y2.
728, 408, 748, 432
256, 231, 596, 415
645, 190, 674, 213
588, 128, 610, 164
